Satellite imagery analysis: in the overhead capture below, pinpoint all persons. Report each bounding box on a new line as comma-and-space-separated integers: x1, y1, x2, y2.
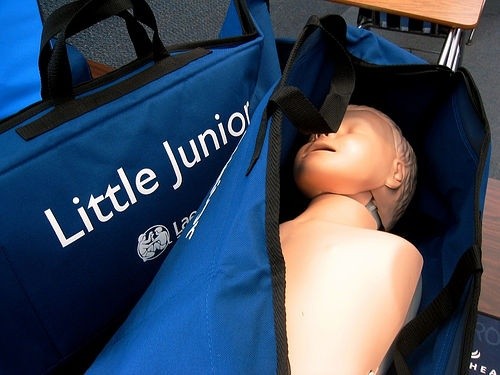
277, 104, 426, 375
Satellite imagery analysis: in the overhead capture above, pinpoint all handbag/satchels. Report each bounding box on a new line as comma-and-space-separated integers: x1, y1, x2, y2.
84, 12, 492, 375
0, 0, 282, 375
